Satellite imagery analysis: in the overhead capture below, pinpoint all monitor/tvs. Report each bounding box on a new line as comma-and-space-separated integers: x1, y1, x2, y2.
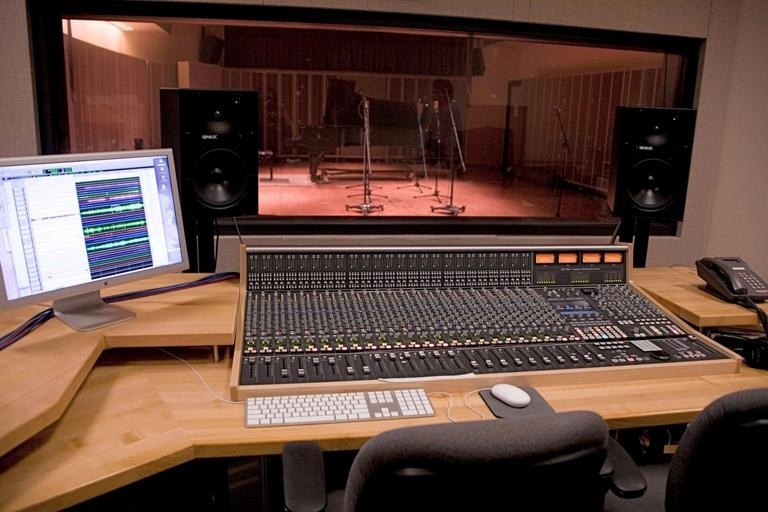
0, 148, 191, 331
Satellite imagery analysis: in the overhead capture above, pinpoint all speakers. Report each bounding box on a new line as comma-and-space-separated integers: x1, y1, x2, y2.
160, 88, 259, 220
607, 106, 696, 221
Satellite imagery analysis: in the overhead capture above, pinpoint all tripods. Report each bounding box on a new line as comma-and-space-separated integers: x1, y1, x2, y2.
414, 110, 451, 203
431, 125, 465, 216
345, 101, 388, 217
397, 103, 432, 193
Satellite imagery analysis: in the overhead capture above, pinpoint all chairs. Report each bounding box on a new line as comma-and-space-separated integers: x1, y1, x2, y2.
281, 410, 647, 512
603, 388, 768, 512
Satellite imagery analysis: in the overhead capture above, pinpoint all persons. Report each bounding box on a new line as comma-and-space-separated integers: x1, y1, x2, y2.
430, 78, 462, 136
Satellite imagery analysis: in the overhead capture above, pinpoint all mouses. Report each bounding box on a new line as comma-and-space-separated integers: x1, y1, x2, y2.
491, 383, 531, 408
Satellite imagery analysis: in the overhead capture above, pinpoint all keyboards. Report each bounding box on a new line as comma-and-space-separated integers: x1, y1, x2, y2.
244, 388, 436, 427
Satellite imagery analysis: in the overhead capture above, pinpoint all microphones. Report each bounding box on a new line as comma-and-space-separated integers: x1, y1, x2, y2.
358, 83, 371, 101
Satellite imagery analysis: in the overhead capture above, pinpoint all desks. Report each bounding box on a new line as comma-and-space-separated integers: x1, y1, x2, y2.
1, 263, 767, 511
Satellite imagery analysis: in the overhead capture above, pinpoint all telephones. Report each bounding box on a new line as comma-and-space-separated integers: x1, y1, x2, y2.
695, 256, 768, 303
